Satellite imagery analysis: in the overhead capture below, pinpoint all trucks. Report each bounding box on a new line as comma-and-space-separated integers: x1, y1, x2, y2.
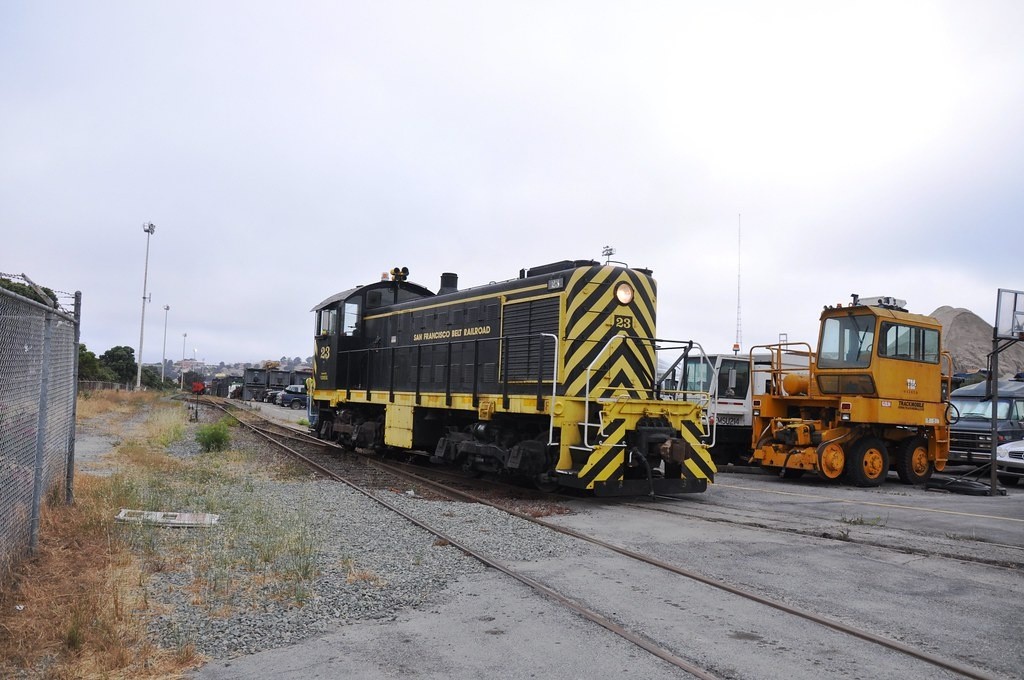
656, 344, 816, 466
948, 372, 1023, 474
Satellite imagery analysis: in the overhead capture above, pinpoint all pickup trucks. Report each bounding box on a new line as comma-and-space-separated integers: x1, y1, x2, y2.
281, 393, 307, 410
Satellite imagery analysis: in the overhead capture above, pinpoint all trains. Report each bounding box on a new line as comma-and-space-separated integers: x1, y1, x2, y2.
306, 266, 718, 496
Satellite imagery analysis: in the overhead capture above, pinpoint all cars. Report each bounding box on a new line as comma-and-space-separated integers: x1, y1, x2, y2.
995, 439, 1024, 486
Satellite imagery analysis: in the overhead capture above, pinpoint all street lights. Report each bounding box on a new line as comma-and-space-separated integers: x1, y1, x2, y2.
180, 333, 186, 390
136, 222, 156, 387
161, 305, 170, 384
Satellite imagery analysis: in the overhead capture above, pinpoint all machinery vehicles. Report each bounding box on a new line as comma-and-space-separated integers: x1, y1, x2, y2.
749, 293, 963, 488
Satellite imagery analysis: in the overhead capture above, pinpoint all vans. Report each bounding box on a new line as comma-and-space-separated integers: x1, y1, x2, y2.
275, 384, 306, 407
